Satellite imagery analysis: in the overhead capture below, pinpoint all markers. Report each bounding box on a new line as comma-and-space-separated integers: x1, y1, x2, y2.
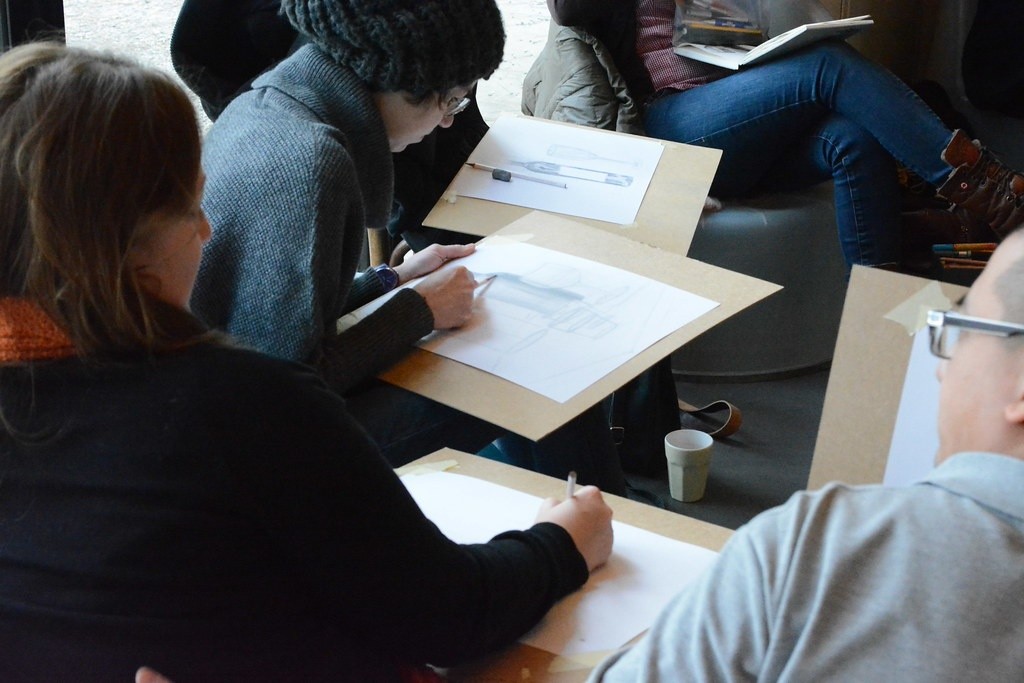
678, 0, 749, 23
701, 19, 743, 28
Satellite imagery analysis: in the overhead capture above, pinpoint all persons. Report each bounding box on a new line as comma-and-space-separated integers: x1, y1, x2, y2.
159, 0, 1024, 474
588, 225, 1024, 683
0, 38, 617, 683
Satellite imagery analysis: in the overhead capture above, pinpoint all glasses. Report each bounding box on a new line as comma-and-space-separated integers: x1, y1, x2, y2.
439, 89, 472, 118
925, 309, 1024, 360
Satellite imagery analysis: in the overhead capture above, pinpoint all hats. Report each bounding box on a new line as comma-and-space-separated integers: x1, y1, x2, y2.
280, 0, 507, 90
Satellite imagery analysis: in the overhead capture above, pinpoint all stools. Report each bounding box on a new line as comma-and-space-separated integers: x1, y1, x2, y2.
669, 179, 849, 380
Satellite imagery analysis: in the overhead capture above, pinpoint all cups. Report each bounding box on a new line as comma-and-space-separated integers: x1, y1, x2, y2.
665, 429, 714, 503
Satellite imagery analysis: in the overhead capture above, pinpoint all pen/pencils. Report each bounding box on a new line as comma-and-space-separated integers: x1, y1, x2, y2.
689, 23, 761, 33
478, 274, 496, 286
567, 472, 577, 499
933, 241, 998, 269
464, 160, 566, 188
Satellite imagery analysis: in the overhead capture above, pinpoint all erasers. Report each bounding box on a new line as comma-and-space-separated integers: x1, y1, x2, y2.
492, 169, 512, 182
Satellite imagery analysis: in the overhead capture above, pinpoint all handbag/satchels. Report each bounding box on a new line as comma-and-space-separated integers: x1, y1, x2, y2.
599, 355, 744, 466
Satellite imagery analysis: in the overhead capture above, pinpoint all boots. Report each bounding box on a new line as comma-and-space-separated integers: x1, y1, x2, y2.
938, 128, 1024, 236
